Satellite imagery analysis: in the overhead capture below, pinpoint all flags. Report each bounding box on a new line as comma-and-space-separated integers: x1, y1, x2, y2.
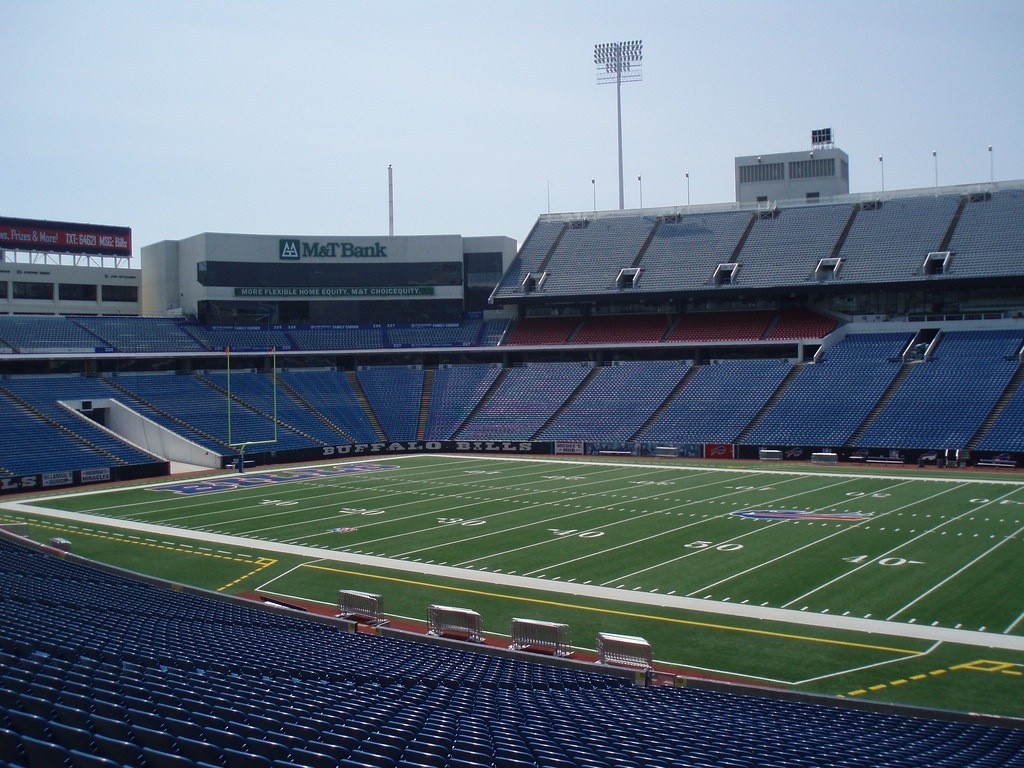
270, 348, 274, 358
225, 346, 228, 357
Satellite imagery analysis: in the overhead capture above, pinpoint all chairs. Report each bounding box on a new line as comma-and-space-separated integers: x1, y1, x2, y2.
0, 530, 1024, 768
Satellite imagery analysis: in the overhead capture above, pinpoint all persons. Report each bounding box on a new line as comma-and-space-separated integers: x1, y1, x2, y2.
643, 667, 656, 681
